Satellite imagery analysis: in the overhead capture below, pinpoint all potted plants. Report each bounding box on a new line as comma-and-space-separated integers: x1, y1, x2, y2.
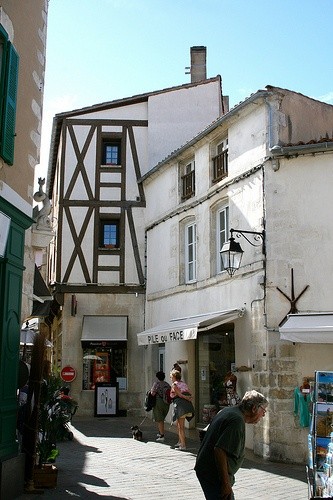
33, 372, 77, 489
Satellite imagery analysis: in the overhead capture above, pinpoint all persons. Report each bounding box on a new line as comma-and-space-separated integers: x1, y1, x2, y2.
194, 390, 269, 500
151, 370, 193, 450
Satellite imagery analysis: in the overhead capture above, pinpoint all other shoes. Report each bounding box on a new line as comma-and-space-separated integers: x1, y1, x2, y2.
174, 442, 186, 451
156, 430, 165, 441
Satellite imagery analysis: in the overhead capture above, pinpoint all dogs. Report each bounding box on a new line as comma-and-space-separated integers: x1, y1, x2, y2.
131, 425, 142, 441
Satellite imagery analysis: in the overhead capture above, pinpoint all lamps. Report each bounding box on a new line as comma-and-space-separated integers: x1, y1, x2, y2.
219, 228, 267, 278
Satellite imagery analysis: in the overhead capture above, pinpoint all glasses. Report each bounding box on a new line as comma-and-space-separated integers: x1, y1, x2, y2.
260, 406, 267, 414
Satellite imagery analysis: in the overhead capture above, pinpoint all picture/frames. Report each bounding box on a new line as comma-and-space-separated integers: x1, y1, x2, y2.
93, 382, 120, 418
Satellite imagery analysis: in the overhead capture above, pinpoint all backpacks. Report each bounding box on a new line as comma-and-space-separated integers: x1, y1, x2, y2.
156, 385, 173, 405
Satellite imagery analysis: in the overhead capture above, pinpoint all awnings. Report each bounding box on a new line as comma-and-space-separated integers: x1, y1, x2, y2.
80, 316, 130, 340
279, 312, 333, 344
137, 309, 237, 346
